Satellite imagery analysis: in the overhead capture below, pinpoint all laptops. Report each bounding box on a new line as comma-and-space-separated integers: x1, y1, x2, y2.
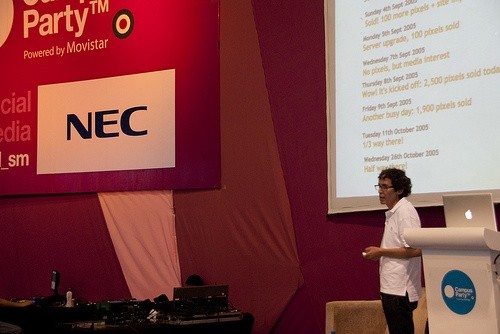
442, 193, 498, 231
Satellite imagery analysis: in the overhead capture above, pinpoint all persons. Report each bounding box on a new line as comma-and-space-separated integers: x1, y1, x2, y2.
185, 275, 204, 287
362, 168, 422, 334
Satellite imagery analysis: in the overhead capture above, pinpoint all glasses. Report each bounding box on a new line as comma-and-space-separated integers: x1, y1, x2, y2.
376, 184, 395, 191
